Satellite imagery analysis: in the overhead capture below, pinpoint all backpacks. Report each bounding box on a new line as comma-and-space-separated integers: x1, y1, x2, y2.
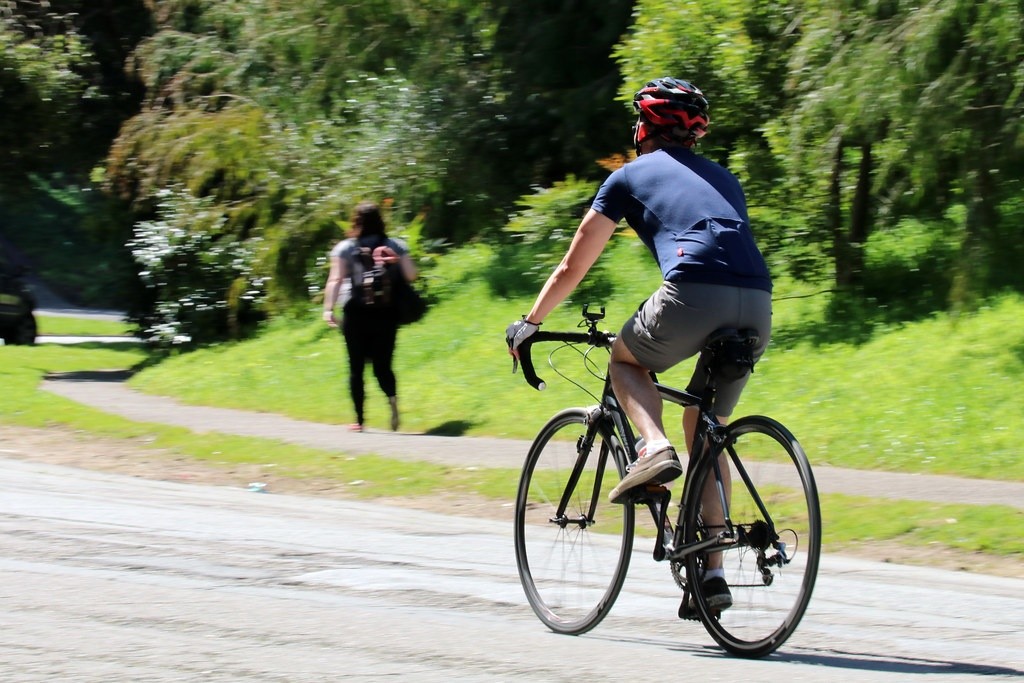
348, 247, 429, 334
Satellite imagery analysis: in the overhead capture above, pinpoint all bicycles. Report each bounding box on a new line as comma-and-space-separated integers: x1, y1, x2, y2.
506, 303, 822, 659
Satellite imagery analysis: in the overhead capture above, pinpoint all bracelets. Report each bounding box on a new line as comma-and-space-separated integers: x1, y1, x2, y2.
323, 309, 334, 312
523, 320, 544, 326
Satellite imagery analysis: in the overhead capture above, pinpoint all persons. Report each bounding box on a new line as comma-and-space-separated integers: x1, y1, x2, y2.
323, 203, 421, 435
505, 73, 774, 623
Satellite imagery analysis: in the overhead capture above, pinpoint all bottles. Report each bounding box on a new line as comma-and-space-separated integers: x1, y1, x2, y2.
634, 434, 674, 490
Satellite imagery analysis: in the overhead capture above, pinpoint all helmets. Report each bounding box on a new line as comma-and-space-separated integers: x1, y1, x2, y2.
633, 76, 712, 149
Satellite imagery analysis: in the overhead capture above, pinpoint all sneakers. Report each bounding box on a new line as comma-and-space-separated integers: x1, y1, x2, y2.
608, 445, 682, 504
687, 576, 733, 615
